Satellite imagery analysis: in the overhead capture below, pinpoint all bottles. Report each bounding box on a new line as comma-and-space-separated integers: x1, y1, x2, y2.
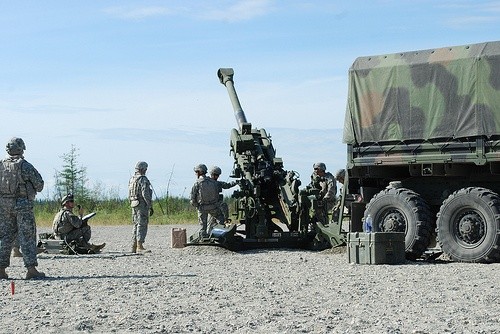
365, 215, 372, 232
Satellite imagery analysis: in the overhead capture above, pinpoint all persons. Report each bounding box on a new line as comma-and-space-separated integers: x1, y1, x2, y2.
0, 136, 45, 279
128, 160, 154, 254
52, 195, 106, 252
335, 168, 345, 183
190, 163, 225, 235
306, 162, 336, 228
207, 166, 237, 230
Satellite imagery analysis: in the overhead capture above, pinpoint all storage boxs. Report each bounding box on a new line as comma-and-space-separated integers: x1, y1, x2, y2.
172, 227, 186, 248
346, 231, 405, 266
43, 240, 66, 255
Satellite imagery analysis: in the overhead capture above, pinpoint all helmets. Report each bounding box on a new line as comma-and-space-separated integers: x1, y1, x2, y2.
193, 163, 208, 173
61, 195, 74, 205
313, 163, 326, 170
210, 166, 221, 174
337, 169, 345, 180
6, 137, 26, 154
134, 161, 148, 169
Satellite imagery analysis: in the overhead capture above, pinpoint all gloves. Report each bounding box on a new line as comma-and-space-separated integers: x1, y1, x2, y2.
150, 207, 154, 216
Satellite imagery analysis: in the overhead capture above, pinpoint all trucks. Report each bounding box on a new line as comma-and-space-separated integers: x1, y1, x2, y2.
341, 41, 500, 264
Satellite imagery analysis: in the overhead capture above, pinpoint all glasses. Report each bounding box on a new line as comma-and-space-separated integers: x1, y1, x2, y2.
68, 200, 74, 203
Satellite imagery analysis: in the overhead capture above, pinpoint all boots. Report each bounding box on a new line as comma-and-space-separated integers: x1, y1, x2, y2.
91, 242, 106, 253
0, 268, 8, 279
26, 266, 45, 279
131, 245, 151, 253
13, 247, 23, 257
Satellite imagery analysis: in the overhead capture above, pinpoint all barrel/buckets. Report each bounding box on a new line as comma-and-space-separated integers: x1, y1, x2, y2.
172, 228, 187, 247
